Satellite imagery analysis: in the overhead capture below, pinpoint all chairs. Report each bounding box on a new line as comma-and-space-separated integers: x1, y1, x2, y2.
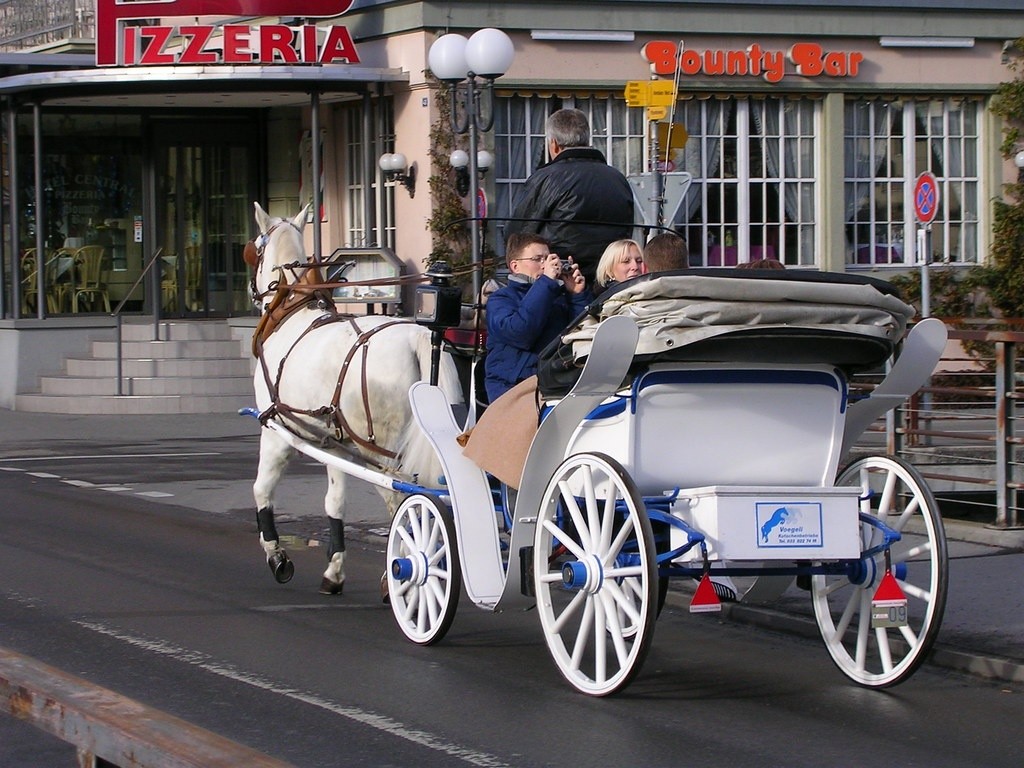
20, 245, 112, 316
161, 246, 202, 312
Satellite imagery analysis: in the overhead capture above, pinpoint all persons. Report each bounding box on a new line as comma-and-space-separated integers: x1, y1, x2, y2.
502, 106, 635, 283
484, 231, 788, 403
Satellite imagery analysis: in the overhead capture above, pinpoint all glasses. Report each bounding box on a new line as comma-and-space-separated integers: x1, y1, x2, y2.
515, 256, 549, 263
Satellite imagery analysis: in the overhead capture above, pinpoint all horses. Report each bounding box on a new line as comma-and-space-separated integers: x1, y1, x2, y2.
247, 201, 464, 605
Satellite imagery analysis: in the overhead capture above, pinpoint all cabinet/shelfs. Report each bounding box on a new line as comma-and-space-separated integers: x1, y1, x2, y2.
84, 226, 142, 270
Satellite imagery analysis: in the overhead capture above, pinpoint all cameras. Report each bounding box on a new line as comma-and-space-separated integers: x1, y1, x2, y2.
559, 260, 573, 274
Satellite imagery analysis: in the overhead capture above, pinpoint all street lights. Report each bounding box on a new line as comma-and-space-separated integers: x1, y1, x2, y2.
429, 23, 518, 304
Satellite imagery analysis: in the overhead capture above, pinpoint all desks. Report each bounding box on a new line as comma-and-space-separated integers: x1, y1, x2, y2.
3, 257, 76, 282
710, 243, 776, 265
851, 242, 918, 265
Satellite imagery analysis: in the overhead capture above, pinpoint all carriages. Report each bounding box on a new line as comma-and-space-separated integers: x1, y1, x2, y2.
240, 196, 948, 700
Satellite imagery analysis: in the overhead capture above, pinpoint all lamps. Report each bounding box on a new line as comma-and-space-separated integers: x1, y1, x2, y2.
1014, 151, 1024, 186
379, 152, 415, 199
450, 149, 492, 198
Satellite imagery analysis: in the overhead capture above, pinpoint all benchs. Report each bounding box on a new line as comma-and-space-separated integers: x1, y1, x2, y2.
441, 279, 508, 357
